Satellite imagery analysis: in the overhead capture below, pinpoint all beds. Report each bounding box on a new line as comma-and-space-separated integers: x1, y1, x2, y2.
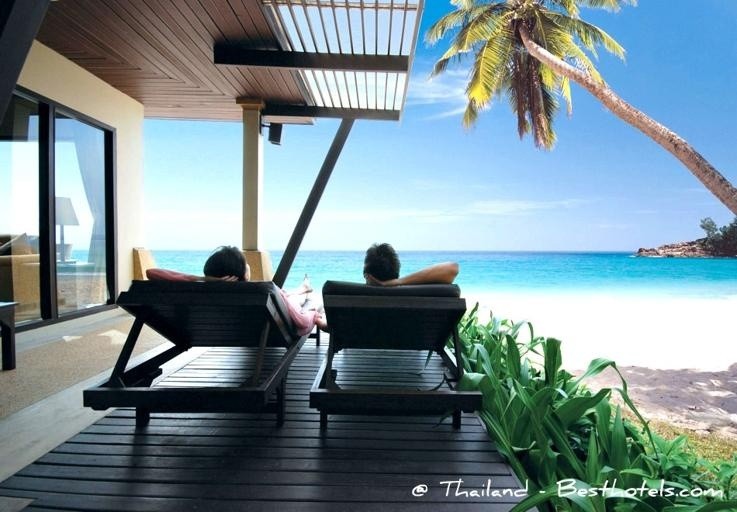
0, 302, 19, 370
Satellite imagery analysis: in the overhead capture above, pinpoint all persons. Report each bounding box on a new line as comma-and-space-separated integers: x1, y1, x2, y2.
144, 244, 316, 337
313, 239, 460, 335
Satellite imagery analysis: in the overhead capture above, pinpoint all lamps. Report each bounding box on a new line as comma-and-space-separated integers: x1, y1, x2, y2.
259, 114, 283, 146
55, 196, 80, 263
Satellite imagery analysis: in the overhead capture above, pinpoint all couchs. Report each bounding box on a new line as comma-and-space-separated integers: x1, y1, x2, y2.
0, 234, 40, 322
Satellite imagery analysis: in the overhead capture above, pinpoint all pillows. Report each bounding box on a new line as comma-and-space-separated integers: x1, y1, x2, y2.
0, 232, 33, 256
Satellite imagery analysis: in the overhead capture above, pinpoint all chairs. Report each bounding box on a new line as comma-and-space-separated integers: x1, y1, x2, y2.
81, 278, 483, 433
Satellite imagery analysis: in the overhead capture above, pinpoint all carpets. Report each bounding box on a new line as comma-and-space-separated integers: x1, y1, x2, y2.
0, 316, 168, 419
